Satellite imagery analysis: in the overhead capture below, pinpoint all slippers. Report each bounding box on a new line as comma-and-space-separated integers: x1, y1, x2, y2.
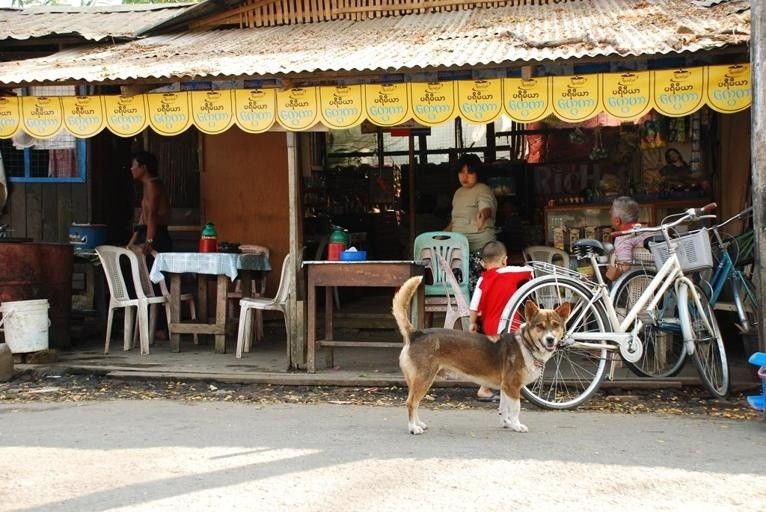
474, 392, 501, 401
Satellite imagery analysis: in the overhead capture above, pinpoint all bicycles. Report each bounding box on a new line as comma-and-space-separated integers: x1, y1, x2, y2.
497, 202, 732, 410
610, 206, 758, 378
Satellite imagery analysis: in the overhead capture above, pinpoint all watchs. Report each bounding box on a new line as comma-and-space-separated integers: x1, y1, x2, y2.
147, 237, 154, 243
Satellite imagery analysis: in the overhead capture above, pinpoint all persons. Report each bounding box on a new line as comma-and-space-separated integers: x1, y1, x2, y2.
662, 149, 691, 176
126, 150, 169, 339
583, 195, 666, 361
468, 240, 535, 403
433, 153, 499, 332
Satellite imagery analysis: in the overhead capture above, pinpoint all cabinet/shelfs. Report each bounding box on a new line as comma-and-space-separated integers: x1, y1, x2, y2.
541, 196, 719, 288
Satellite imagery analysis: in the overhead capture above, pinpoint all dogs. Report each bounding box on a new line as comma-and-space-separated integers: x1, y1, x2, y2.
392, 275, 571, 435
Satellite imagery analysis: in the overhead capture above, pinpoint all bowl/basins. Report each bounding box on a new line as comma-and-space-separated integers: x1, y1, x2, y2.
597, 256, 608, 264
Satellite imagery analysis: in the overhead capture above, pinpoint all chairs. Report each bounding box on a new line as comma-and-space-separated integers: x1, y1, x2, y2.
522, 249, 589, 346
313, 237, 342, 311
126, 244, 200, 346
413, 232, 474, 335
96, 246, 172, 356
226, 245, 271, 342
235, 246, 307, 361
612, 245, 675, 371
